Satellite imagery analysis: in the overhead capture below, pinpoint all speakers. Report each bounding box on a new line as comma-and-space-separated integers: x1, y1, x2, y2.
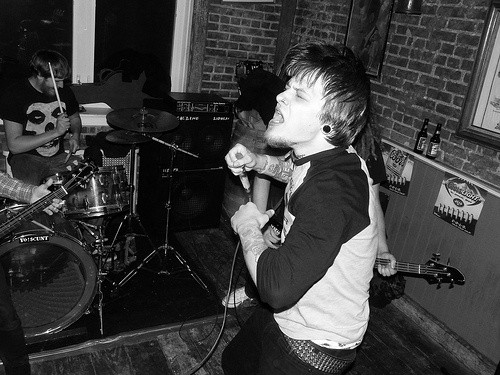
139, 93, 233, 234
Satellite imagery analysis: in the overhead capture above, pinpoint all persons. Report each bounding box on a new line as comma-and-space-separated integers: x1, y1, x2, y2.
0, 50, 117, 263
0, 171, 62, 375
222, 107, 397, 308
221, 41, 379, 375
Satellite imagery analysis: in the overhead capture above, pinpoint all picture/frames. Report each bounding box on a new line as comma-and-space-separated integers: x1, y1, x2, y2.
454, 0, 499, 151
343, 0, 395, 79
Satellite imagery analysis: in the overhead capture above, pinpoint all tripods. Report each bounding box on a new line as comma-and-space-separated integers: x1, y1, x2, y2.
99, 132, 217, 305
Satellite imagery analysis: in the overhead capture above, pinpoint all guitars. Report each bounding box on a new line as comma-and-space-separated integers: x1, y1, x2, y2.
0, 157, 99, 239
374, 253, 464, 284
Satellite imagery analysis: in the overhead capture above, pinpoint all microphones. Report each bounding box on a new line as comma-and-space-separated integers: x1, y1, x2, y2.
236, 152, 252, 195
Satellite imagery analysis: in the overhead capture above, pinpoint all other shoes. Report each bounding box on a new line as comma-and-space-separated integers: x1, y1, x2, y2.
82, 241, 117, 262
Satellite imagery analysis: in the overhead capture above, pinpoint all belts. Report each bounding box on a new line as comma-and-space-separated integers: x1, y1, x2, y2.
284, 334, 356, 374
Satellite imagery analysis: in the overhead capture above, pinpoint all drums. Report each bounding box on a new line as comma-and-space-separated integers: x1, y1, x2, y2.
44, 164, 123, 219
0, 202, 97, 338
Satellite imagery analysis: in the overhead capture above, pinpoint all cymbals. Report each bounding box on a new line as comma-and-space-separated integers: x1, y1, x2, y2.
106, 131, 145, 142
106, 108, 178, 133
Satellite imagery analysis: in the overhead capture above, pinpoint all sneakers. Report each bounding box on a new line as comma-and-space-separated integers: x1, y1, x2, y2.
222, 286, 253, 308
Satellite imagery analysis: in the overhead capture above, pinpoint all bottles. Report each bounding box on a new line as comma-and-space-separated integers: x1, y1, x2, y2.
426, 123, 442, 160
413, 122, 428, 154
